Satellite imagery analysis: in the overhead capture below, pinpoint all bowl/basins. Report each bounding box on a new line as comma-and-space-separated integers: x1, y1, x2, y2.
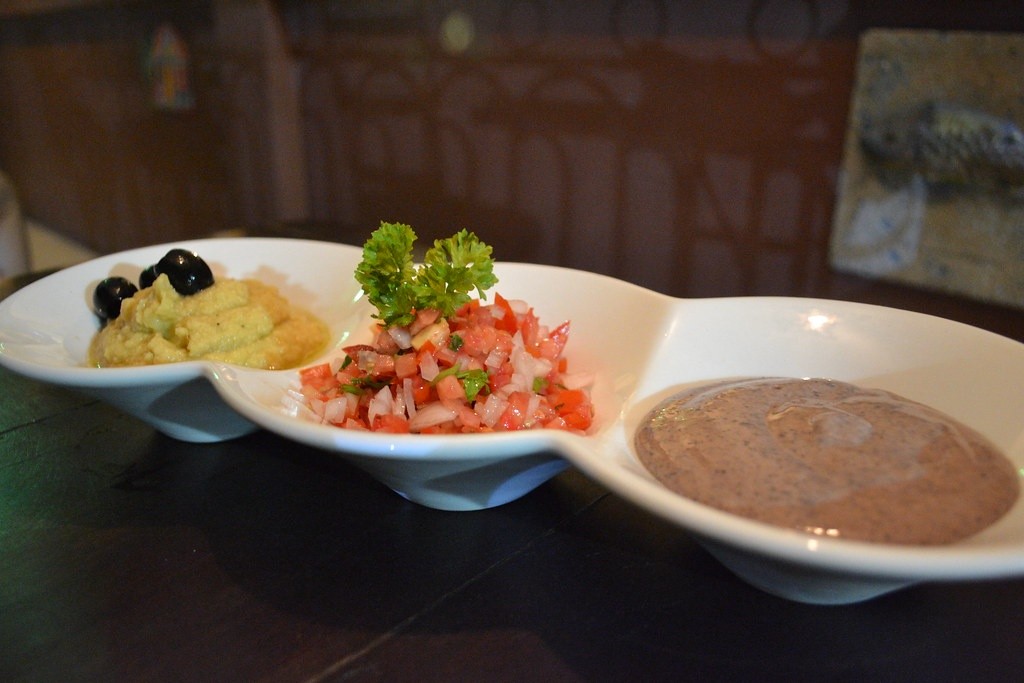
0, 236, 1024, 606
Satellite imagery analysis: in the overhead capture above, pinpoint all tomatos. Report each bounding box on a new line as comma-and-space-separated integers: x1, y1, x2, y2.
296, 294, 593, 436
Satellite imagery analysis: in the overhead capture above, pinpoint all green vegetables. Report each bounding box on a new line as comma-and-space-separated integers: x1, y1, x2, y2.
333, 220, 569, 405
